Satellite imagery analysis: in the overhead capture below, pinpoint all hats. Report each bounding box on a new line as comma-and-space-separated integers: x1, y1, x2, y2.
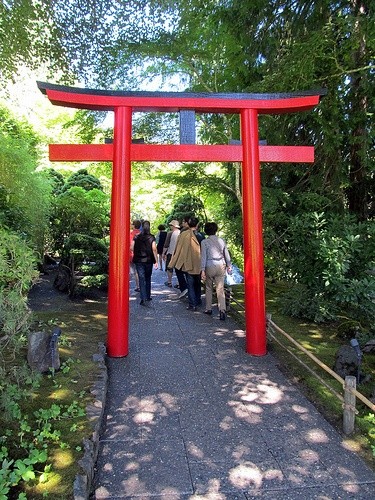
168, 220, 180, 228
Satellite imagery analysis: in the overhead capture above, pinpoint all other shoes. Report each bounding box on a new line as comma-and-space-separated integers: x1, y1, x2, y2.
178, 289, 188, 298
135, 288, 140, 291
146, 298, 152, 301
204, 310, 212, 314
220, 312, 225, 320
186, 305, 195, 310
140, 300, 145, 305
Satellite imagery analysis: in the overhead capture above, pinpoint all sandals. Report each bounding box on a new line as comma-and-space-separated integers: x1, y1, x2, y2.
164, 281, 172, 286
174, 285, 180, 288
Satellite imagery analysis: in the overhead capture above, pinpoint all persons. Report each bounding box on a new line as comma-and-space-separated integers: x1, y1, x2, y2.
201, 223, 232, 319
129, 218, 205, 310
